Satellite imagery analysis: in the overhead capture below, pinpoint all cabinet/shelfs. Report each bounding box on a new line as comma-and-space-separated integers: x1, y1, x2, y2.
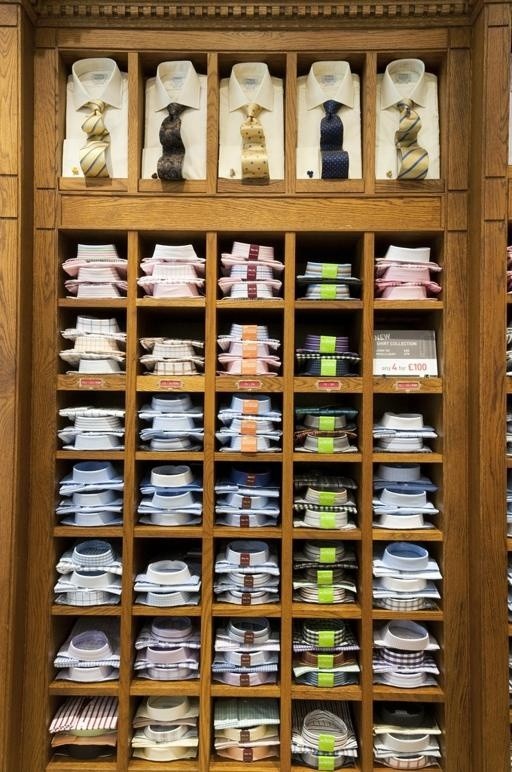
1, 0, 512, 772
